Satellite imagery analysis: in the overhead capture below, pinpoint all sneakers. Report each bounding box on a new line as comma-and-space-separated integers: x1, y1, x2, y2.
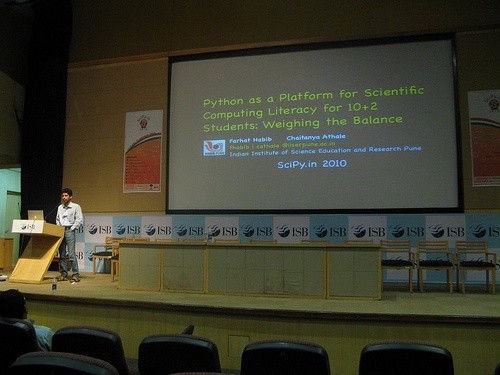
56, 275, 67, 281
72, 274, 80, 282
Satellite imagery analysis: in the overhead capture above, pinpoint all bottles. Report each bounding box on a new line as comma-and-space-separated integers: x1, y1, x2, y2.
51, 278, 57, 292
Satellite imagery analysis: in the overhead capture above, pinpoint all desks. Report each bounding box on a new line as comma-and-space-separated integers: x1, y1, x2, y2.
118, 241, 382, 302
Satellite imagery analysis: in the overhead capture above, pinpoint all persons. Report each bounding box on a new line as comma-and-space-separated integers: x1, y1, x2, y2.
56, 188, 83, 282
0, 289, 55, 352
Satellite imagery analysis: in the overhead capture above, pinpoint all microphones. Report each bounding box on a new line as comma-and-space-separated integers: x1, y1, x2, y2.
44, 205, 58, 219
17, 203, 21, 219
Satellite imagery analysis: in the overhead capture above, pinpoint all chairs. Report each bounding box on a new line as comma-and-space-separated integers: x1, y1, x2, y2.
0, 316, 222, 375
359, 342, 454, 375
415, 240, 455, 293
92, 237, 374, 282
454, 240, 497, 295
380, 239, 416, 293
241, 340, 330, 375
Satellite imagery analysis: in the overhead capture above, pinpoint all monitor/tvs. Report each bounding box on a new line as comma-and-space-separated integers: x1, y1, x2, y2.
28, 209, 44, 220
